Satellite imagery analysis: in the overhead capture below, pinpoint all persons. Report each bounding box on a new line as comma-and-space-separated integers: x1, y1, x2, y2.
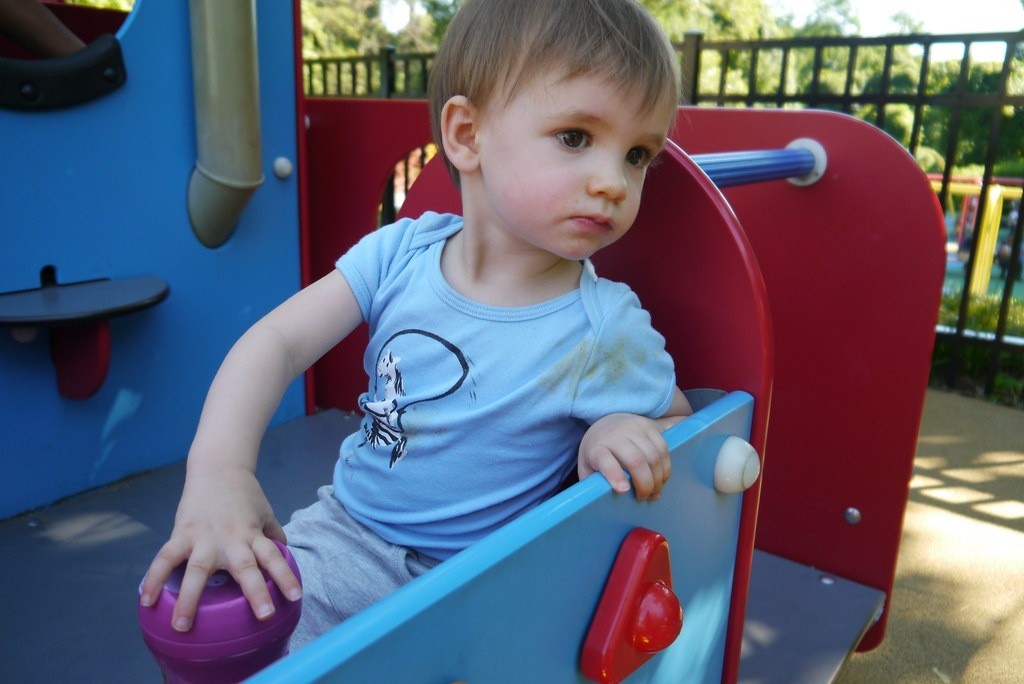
140, 0, 692, 654
